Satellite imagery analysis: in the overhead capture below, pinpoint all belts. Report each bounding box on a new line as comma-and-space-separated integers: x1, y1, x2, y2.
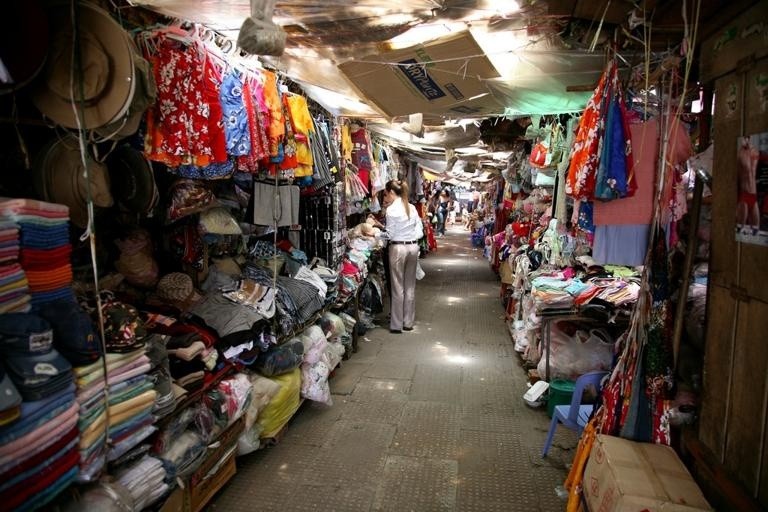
388, 240, 420, 245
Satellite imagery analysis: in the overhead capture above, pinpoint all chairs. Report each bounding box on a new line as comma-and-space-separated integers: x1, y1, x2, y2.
542, 371, 612, 458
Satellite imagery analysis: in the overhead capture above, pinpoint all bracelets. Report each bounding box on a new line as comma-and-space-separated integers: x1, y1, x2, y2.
373, 233, 375, 237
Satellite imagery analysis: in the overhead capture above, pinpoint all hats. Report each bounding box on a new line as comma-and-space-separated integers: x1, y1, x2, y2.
0, 0, 160, 236
0, 294, 150, 430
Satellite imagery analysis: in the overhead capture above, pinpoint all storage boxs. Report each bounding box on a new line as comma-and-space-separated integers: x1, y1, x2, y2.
155, 415, 246, 512
582, 434, 714, 512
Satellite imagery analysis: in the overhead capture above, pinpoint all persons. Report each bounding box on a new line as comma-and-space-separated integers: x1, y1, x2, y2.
462, 203, 470, 230
362, 180, 424, 333
368, 191, 391, 323
435, 189, 450, 237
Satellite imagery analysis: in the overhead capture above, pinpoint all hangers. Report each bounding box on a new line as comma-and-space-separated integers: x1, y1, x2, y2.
128, 14, 399, 156
566, 86, 690, 137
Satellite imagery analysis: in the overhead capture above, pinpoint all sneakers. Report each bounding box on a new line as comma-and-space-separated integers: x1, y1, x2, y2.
390, 325, 414, 335
